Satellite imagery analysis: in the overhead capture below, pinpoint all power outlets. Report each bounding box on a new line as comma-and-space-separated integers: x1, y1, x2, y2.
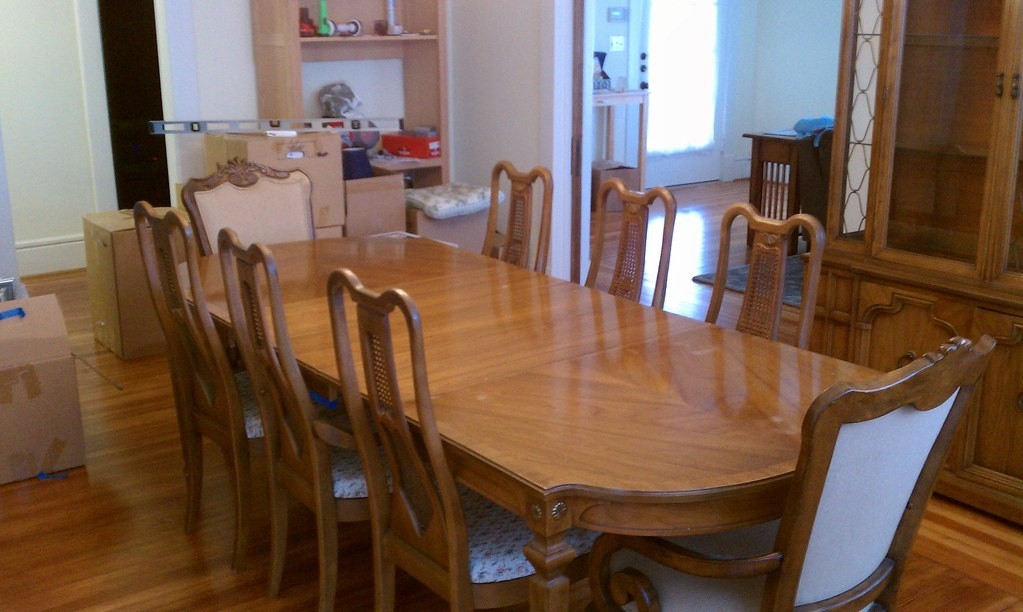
0, 281, 14, 303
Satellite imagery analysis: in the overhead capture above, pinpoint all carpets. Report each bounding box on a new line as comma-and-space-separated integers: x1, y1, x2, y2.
691, 245, 807, 306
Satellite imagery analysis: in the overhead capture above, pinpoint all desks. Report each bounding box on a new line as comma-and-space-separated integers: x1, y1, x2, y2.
175, 228, 897, 612
592, 86, 652, 194
740, 128, 830, 252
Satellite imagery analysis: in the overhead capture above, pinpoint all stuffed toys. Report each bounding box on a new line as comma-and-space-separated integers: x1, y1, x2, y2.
321, 82, 379, 148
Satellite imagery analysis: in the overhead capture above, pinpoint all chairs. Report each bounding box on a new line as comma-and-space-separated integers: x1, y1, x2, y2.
578, 177, 679, 310
589, 326, 1001, 612
214, 227, 396, 612
181, 150, 322, 260
700, 200, 829, 351
321, 263, 593, 612
481, 156, 557, 275
119, 198, 275, 576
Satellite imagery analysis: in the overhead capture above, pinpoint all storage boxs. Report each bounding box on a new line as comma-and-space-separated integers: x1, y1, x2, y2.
383, 132, 440, 157
592, 159, 640, 213
407, 196, 496, 251
0, 291, 91, 484
346, 162, 409, 237
80, 205, 202, 359
203, 126, 352, 228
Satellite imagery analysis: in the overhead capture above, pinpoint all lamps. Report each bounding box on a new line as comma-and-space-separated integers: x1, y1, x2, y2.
342, 147, 373, 179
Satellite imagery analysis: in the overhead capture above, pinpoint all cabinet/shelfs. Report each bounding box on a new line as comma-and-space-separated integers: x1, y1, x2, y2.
802, 0, 1023, 531
253, 0, 453, 186
